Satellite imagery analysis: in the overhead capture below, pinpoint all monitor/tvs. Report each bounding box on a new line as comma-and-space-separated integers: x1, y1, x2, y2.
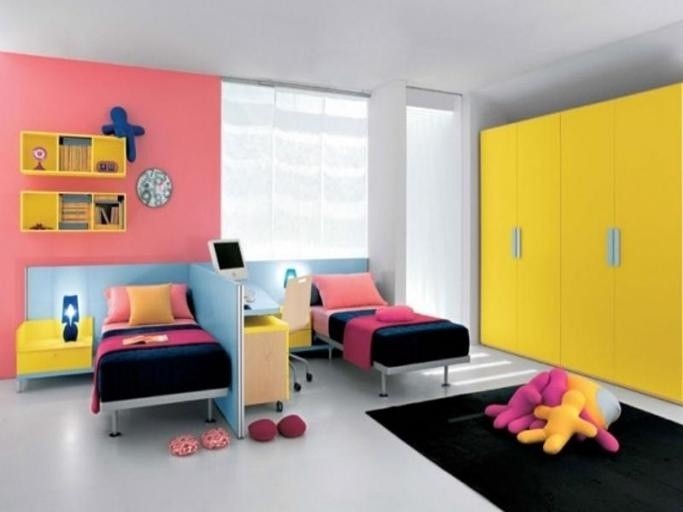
122, 334, 167, 344
59, 137, 91, 172
59, 193, 123, 230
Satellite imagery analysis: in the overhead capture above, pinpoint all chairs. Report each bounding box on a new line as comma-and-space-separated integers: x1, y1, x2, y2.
280, 276, 312, 391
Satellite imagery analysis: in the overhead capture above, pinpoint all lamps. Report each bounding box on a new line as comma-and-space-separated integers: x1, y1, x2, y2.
61, 295, 79, 341
280, 276, 312, 391
284, 268, 298, 288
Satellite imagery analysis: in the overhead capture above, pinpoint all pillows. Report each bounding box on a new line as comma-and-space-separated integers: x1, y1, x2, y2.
376, 305, 415, 321
312, 272, 388, 311
103, 283, 194, 324
310, 283, 323, 306
126, 282, 176, 326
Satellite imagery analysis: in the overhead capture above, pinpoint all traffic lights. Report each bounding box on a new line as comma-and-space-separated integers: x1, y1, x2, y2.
376, 305, 415, 321
103, 283, 194, 324
310, 283, 323, 306
312, 272, 388, 311
126, 282, 176, 326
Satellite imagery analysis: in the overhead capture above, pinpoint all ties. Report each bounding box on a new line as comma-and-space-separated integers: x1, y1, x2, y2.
208, 238, 247, 281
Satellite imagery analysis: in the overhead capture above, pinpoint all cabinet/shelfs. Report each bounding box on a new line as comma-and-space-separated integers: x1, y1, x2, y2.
20, 130, 127, 178
20, 190, 127, 233
479, 111, 561, 367
560, 81, 682, 406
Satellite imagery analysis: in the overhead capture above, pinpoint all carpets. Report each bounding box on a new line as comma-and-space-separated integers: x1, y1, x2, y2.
365, 384, 683, 512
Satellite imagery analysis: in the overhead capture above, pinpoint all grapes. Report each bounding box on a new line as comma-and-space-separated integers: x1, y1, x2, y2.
16, 317, 94, 392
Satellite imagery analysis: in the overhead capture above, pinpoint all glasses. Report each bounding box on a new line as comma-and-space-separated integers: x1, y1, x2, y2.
284, 268, 298, 288
61, 295, 79, 341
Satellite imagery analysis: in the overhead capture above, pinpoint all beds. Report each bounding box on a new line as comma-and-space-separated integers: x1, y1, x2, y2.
311, 304, 470, 397
96, 318, 231, 438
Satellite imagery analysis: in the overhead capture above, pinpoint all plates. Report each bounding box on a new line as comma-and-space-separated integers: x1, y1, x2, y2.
20, 190, 127, 233
20, 130, 127, 178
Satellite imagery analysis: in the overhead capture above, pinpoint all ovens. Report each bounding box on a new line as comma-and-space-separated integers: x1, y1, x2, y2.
311, 304, 470, 397
96, 318, 231, 438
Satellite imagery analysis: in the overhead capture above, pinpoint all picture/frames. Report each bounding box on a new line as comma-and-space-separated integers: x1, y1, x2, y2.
242, 284, 289, 411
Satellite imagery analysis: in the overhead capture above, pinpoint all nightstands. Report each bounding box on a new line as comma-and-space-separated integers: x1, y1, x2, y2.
16, 317, 94, 392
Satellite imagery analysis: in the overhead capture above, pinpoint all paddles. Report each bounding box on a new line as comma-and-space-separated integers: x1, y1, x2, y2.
365, 384, 683, 512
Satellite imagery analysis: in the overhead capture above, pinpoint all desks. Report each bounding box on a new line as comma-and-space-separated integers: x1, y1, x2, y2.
242, 284, 289, 411
